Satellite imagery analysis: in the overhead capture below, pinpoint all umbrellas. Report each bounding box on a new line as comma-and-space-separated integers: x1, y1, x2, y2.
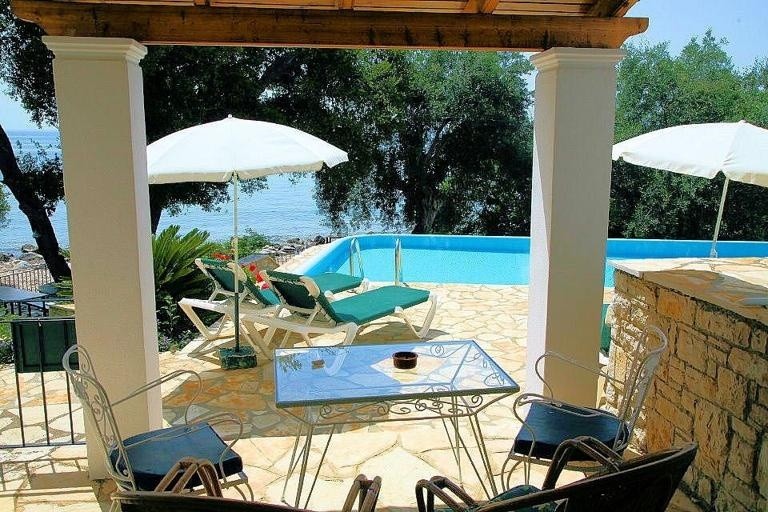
146, 114, 350, 352
611, 119, 768, 259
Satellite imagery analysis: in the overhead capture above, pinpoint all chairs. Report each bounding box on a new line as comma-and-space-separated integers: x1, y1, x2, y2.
177, 256, 441, 378
107, 456, 382, 512
500, 325, 669, 491
60, 342, 256, 512
415, 442, 701, 512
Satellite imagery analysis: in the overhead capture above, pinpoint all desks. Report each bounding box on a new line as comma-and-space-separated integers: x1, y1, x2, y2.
273, 339, 521, 508
